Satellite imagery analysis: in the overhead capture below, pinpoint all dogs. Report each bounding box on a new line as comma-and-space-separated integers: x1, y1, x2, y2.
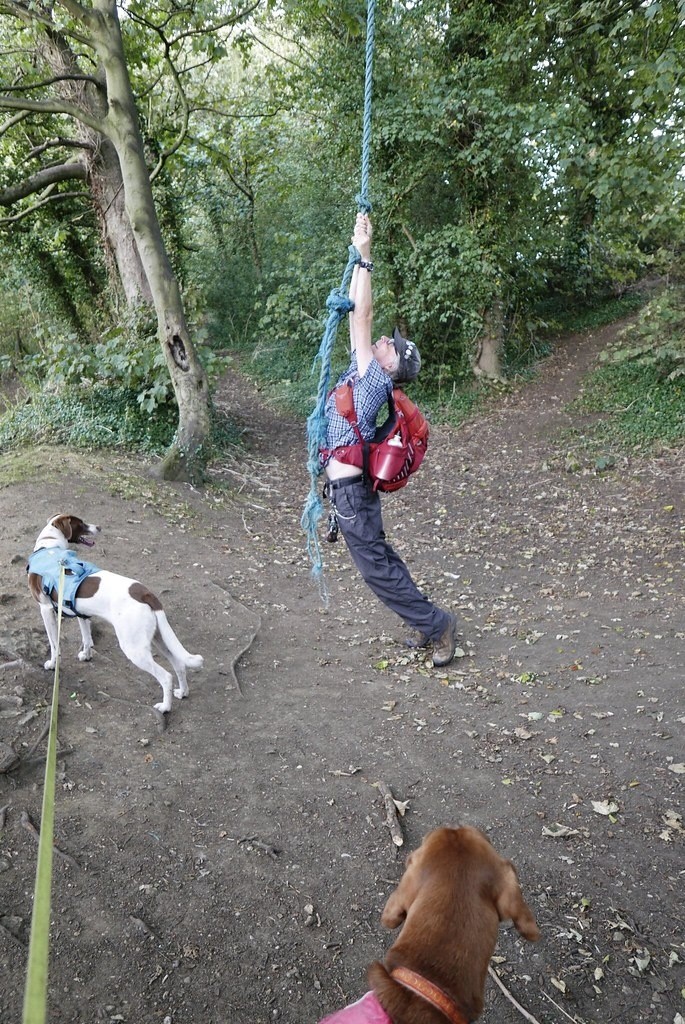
27, 512, 203, 715
315, 824, 540, 1024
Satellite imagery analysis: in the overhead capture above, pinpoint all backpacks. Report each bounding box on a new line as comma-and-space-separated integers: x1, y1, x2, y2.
327, 372, 430, 493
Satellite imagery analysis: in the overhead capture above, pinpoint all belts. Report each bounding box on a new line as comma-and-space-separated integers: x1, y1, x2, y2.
323, 477, 362, 489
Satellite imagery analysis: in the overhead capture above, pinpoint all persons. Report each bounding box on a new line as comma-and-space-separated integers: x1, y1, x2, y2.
321, 214, 457, 665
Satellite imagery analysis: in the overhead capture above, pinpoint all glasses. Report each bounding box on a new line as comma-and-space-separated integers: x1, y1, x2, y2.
386, 339, 398, 357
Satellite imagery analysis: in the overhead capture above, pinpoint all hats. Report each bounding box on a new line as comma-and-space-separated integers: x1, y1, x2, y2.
392, 326, 421, 383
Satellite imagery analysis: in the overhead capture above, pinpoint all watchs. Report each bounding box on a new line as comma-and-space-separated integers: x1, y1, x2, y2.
360, 262, 374, 271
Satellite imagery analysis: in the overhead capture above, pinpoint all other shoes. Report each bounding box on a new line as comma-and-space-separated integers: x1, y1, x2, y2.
433, 613, 456, 666
407, 629, 430, 648
